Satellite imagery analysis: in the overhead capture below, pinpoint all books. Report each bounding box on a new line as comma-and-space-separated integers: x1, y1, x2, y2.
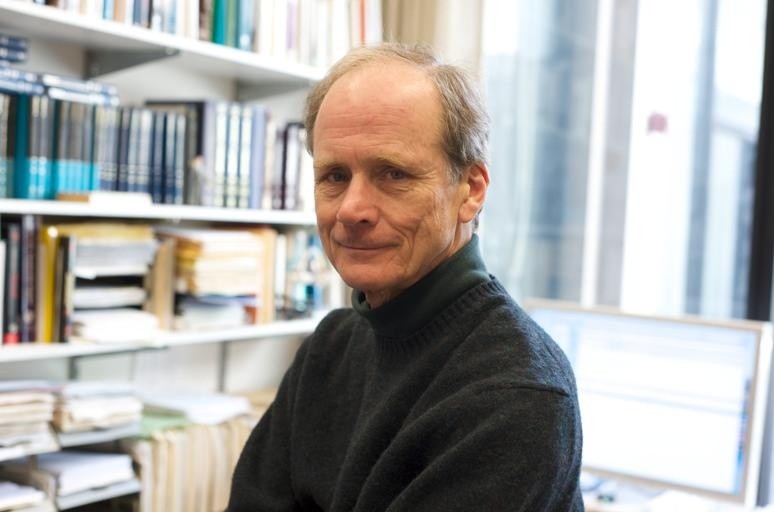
0, 214, 347, 345
2, 379, 279, 511
30, 0, 386, 74
1, 23, 315, 210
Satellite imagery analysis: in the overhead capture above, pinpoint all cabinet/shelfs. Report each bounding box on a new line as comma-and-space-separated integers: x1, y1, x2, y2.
0, 0, 369, 512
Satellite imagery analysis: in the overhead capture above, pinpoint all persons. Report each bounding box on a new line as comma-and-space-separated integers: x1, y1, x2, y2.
224, 44, 585, 512
